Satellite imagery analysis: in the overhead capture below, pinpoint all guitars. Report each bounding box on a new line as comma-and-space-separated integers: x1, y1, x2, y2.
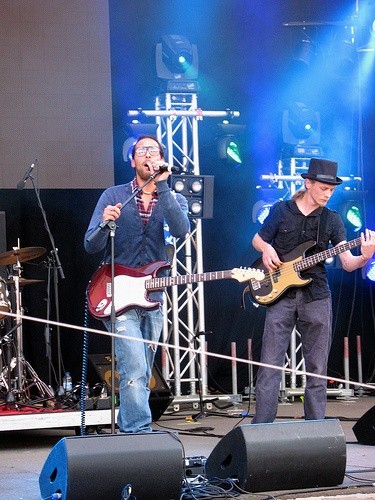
248, 233, 372, 305
88, 260, 265, 322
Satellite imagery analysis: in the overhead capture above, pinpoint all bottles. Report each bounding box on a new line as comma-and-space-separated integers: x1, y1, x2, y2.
101, 383, 107, 398
63, 372, 72, 391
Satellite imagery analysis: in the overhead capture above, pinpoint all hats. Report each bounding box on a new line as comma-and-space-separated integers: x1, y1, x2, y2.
301, 158, 343, 185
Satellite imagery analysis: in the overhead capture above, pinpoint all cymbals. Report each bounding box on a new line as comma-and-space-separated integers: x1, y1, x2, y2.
0, 246, 47, 265
7, 279, 45, 285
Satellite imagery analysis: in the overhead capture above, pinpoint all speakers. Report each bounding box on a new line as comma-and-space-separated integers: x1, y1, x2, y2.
38, 431, 182, 500
73, 354, 174, 422
352, 405, 375, 446
205, 418, 347, 493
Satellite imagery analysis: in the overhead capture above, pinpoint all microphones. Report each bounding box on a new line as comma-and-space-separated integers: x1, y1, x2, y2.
17, 159, 37, 190
159, 166, 179, 171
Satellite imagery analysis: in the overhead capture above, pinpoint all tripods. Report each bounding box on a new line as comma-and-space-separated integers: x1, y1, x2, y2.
0, 171, 76, 413
185, 331, 238, 421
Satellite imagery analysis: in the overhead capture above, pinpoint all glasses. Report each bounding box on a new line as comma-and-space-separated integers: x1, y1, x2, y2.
134, 147, 161, 157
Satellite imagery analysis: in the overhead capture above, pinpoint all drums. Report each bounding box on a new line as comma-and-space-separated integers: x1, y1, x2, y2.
0, 277, 12, 321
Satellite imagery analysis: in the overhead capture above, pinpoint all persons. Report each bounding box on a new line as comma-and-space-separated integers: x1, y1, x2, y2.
83, 136, 190, 434
251, 158, 375, 424
